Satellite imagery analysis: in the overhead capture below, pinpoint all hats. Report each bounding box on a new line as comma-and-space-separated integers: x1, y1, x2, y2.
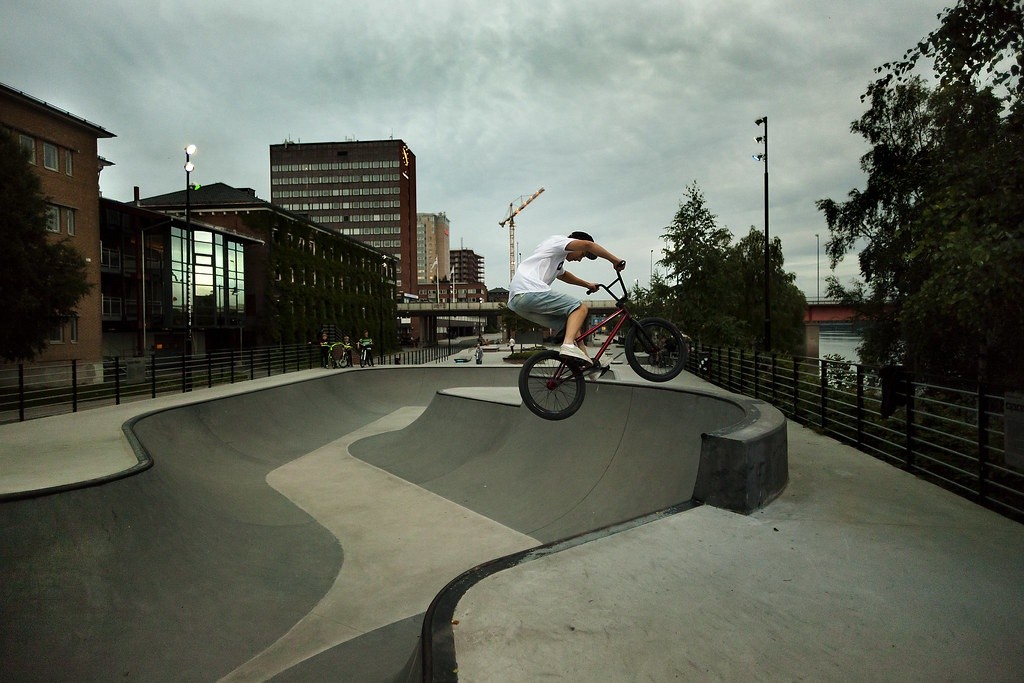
567, 231, 598, 260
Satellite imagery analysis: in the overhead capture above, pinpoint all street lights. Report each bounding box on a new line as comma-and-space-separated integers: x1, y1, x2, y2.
651, 250, 654, 282
479, 296, 483, 337
448, 285, 453, 345
184, 144, 196, 355
752, 116, 771, 352
815, 234, 819, 305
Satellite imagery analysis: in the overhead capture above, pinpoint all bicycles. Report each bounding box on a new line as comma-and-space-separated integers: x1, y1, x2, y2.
517, 260, 690, 422
328, 349, 337, 369
338, 347, 351, 368
359, 344, 372, 367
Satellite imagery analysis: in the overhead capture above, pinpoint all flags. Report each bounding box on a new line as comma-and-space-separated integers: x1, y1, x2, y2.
448, 265, 454, 281
430, 257, 438, 272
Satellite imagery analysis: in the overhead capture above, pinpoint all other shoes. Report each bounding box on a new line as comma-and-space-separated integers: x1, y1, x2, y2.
372, 365, 376, 367
369, 365, 371, 366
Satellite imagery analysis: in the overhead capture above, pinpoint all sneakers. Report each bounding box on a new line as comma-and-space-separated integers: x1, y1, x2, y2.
559, 344, 593, 366
588, 357, 613, 382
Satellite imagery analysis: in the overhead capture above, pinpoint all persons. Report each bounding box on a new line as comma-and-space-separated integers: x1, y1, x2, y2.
509, 336, 515, 354
478, 296, 483, 303
320, 334, 331, 369
342, 336, 353, 367
474, 343, 484, 364
357, 330, 375, 367
478, 334, 488, 345
507, 232, 626, 382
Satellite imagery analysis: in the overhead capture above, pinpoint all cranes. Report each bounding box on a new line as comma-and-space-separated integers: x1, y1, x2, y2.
497, 188, 546, 284
